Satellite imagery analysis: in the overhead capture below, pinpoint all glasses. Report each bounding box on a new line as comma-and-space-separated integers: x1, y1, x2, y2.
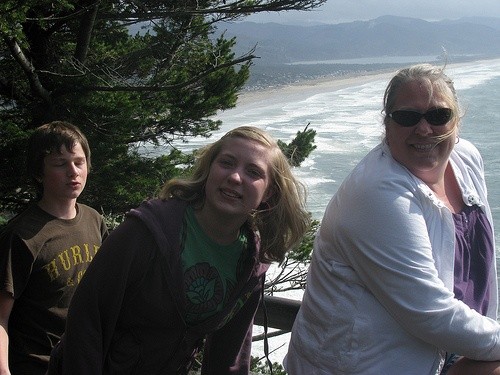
390, 108, 458, 128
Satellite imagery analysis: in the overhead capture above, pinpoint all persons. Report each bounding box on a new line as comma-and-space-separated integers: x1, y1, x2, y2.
44, 126, 310, 375
283, 63, 500, 375
0, 121, 109, 375
